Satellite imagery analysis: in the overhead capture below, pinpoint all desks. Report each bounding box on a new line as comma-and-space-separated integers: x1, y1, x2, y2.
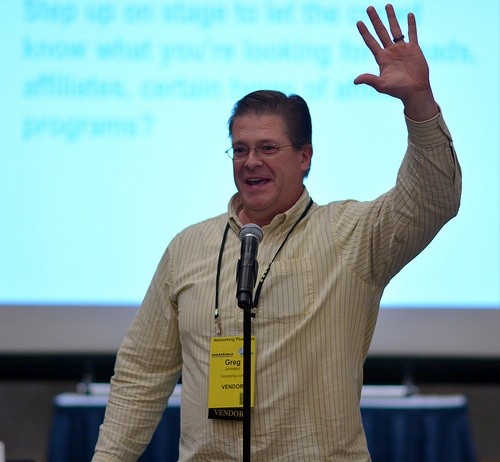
48, 392, 474, 462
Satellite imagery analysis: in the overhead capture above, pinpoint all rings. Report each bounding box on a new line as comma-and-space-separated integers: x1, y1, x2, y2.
393, 35, 405, 43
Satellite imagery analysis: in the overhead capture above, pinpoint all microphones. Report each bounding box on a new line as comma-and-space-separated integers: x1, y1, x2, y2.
237, 222, 265, 309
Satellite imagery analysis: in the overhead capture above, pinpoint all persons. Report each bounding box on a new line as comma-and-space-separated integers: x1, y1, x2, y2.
90, 3, 463, 462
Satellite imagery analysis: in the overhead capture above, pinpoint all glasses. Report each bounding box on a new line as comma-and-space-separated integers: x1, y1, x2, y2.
225, 145, 293, 159
